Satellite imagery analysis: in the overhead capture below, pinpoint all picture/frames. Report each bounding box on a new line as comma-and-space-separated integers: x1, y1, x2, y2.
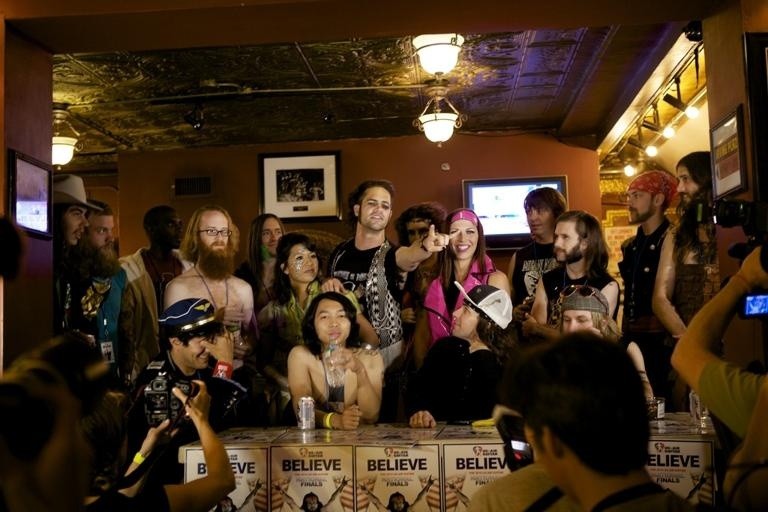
8, 149, 54, 240
256, 150, 344, 222
707, 103, 748, 201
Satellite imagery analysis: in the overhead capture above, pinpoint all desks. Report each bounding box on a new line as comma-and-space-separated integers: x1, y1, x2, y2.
177, 409, 721, 510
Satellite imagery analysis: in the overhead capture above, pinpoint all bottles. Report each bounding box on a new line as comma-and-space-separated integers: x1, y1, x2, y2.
689, 386, 716, 436
326, 336, 347, 396
226, 296, 241, 347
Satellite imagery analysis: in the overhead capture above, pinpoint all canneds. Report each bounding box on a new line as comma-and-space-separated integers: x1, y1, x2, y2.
294, 395, 316, 430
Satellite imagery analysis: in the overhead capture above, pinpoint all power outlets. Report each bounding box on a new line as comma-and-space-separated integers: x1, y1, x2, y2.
412, 85, 468, 147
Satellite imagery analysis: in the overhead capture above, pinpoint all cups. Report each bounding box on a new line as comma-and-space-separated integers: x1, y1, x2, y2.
647, 396, 665, 420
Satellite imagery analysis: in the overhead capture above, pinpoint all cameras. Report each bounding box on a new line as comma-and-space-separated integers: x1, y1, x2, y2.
143, 372, 201, 438
495, 413, 534, 472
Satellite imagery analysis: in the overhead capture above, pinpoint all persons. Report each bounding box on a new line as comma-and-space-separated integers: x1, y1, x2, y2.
464, 454, 586, 511
272, 473, 352, 511
209, 478, 265, 512
499, 327, 702, 512
39, 327, 239, 512
669, 243, 767, 511
446, 479, 471, 508
1, 358, 100, 511
357, 474, 438, 512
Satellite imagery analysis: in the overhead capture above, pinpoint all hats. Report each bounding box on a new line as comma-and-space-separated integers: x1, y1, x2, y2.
456, 281, 512, 330
52, 174, 103, 212
157, 298, 223, 334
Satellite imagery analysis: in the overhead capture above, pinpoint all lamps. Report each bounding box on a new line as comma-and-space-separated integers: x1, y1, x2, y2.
51, 101, 83, 167
622, 104, 699, 177
412, 33, 465, 82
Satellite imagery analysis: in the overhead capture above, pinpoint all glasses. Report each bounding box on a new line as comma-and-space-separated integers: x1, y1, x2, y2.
406, 227, 428, 236
196, 229, 233, 237
